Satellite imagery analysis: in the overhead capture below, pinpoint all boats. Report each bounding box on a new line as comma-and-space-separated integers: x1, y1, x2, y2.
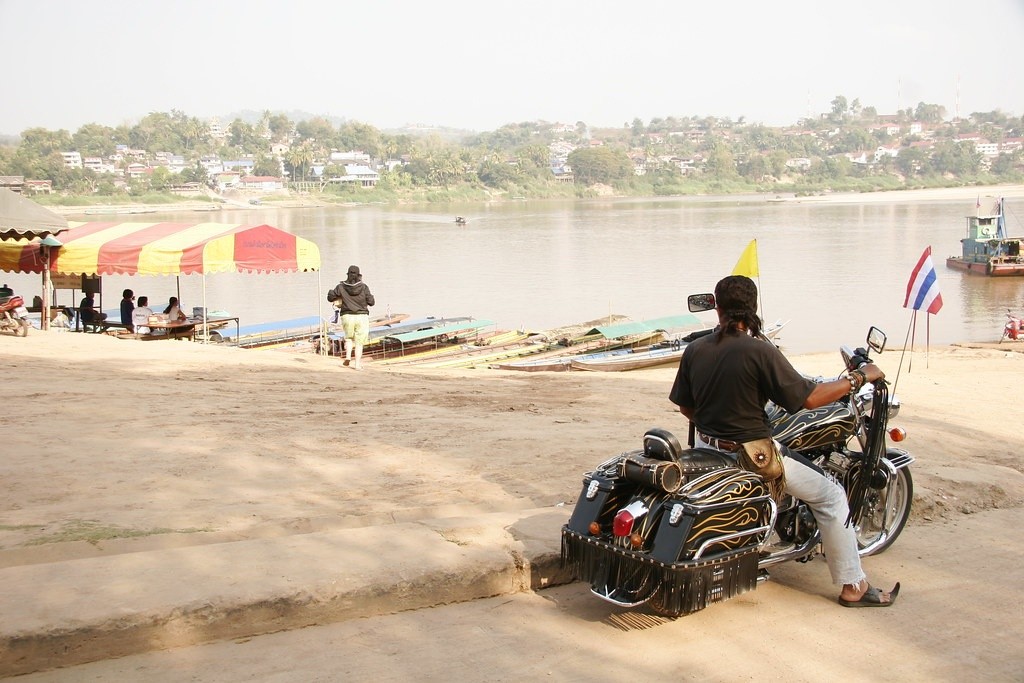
946, 194, 1024, 277
570, 316, 792, 374
312, 311, 669, 373
102, 310, 231, 336
102, 301, 185, 320
207, 314, 326, 347
455, 216, 466, 224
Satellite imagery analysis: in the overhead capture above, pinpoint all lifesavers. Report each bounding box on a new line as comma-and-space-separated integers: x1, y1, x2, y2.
981, 227, 991, 235
674, 339, 681, 351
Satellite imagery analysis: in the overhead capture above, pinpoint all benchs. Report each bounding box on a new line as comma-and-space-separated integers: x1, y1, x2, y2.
82, 320, 134, 334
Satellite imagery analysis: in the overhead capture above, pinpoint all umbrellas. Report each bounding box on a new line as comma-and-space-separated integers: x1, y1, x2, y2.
0, 187, 70, 242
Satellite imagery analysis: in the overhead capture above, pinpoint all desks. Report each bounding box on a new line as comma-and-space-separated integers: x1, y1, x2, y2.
138, 316, 239, 347
50, 308, 63, 322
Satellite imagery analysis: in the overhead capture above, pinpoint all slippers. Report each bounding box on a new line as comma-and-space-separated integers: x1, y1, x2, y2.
839, 581, 900, 607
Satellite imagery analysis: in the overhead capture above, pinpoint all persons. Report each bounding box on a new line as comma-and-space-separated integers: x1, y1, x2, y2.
327, 265, 375, 371
132, 296, 154, 334
163, 297, 186, 321
80, 289, 107, 323
120, 289, 135, 333
669, 275, 901, 609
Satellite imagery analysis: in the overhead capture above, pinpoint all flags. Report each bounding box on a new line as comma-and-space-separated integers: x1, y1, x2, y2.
903, 245, 943, 314
732, 239, 759, 277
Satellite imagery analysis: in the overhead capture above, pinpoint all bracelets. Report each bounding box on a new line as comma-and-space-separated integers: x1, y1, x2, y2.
845, 369, 867, 394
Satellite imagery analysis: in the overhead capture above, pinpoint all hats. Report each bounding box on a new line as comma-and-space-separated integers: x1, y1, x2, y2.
348, 265, 359, 274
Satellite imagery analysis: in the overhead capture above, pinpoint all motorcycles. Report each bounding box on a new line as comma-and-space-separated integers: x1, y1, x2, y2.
558, 291, 915, 619
998, 309, 1024, 344
0, 295, 30, 337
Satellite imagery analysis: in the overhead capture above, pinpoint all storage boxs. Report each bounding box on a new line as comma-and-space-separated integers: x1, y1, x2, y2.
671, 466, 768, 560
567, 450, 645, 535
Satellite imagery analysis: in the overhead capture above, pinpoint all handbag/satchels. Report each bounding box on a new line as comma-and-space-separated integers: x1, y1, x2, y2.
739, 438, 787, 499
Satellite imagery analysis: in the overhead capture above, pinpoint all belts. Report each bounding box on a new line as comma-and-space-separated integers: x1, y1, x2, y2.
699, 433, 742, 452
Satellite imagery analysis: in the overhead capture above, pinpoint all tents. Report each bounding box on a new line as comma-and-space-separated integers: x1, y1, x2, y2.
0, 219, 324, 345
